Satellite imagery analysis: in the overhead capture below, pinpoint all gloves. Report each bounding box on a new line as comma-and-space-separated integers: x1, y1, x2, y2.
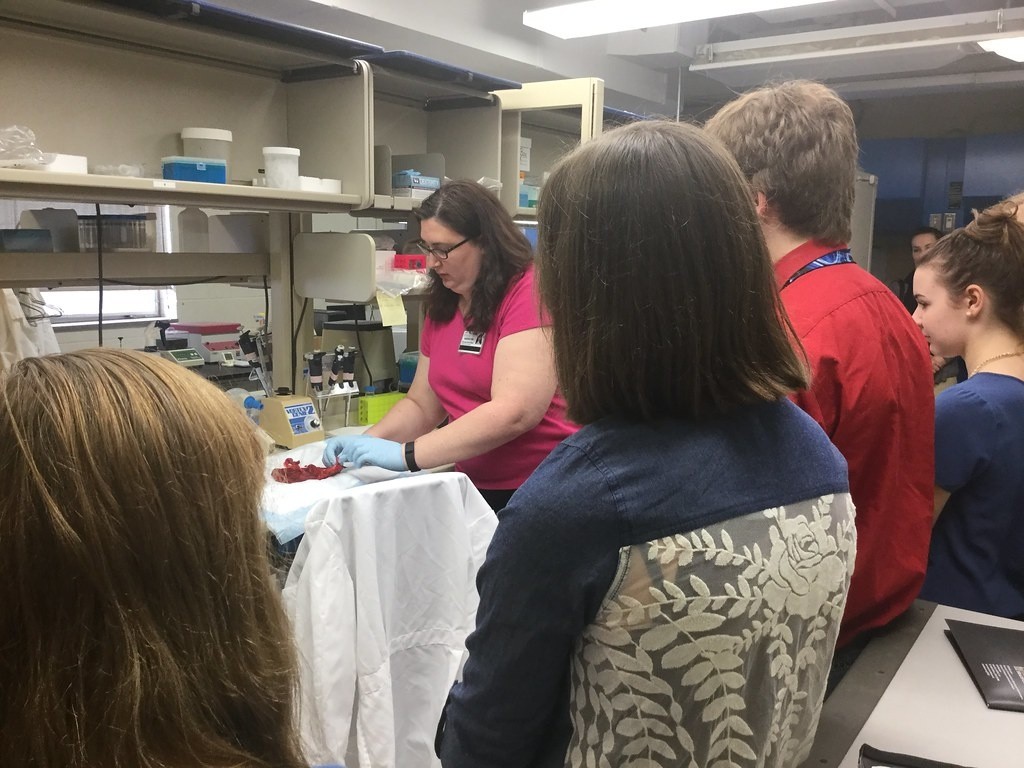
322, 434, 405, 471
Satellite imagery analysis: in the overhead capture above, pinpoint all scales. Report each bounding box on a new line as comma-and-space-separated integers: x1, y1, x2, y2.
158, 348, 205, 368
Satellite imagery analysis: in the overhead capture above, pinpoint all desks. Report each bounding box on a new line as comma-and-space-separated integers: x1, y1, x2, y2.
797, 592, 1024, 768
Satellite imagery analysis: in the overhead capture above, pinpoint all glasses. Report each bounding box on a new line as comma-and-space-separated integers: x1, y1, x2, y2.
417, 237, 470, 260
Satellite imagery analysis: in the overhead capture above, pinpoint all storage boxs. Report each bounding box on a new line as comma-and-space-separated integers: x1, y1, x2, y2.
76, 214, 146, 251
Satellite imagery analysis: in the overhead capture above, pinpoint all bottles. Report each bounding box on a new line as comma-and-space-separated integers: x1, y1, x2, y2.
256, 168, 267, 187
364, 385, 376, 400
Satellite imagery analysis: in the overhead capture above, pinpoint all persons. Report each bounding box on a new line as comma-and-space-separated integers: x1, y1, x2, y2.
431, 116, 864, 768
897, 222, 969, 384
907, 200, 1023, 622
1, 348, 314, 768
701, 78, 939, 708
321, 178, 586, 514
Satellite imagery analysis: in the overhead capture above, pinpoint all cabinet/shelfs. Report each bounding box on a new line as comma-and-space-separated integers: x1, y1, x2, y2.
488, 76, 658, 221
350, 63, 502, 222
0, 1, 375, 406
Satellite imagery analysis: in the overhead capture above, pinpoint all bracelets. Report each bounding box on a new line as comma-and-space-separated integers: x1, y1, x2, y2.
404, 442, 421, 472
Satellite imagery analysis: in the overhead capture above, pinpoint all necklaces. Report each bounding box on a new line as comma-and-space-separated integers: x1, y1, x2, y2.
968, 351, 1024, 379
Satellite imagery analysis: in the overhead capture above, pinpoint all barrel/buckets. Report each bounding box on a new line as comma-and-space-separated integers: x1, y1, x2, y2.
261, 146, 301, 190
180, 127, 233, 184
